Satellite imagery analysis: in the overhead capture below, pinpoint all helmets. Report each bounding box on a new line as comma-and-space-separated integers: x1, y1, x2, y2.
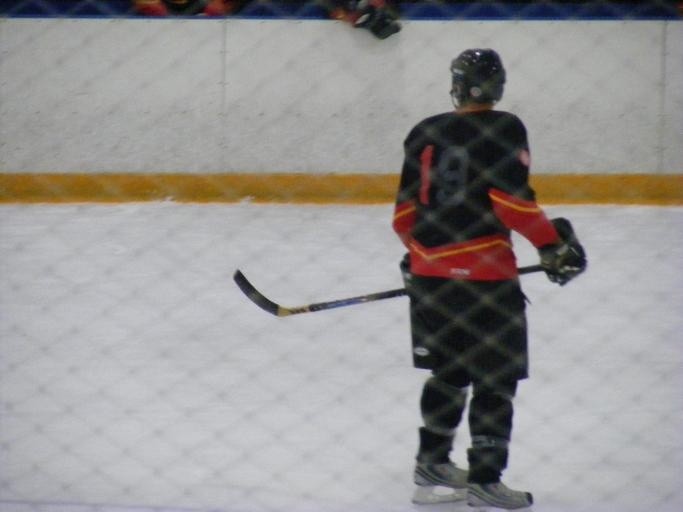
449, 47, 505, 105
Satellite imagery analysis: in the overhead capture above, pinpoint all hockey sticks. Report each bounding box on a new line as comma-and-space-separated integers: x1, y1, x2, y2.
233, 265, 545, 316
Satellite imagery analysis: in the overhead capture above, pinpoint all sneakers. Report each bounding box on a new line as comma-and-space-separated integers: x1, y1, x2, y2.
463, 478, 535, 510
413, 455, 473, 488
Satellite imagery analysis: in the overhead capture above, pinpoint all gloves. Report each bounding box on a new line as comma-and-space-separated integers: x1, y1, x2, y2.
354, 6, 400, 40
399, 253, 414, 301
540, 217, 586, 286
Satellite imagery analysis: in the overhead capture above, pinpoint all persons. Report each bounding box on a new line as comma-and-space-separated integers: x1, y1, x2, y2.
392, 44, 591, 510
135, 1, 239, 21
323, 0, 403, 40
